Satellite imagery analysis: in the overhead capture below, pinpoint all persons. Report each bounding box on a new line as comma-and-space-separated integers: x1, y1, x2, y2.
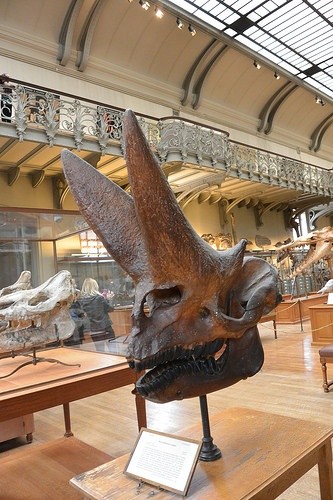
46, 289, 85, 347
80, 277, 118, 354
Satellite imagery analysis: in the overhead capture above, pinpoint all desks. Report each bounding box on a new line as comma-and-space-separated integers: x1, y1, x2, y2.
0, 290, 333, 500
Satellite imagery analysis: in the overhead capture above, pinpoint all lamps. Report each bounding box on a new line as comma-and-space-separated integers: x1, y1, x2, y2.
315, 94, 327, 107
139, 0, 150, 10
274, 72, 280, 80
253, 60, 260, 70
176, 16, 184, 31
155, 6, 164, 20
188, 24, 197, 37
